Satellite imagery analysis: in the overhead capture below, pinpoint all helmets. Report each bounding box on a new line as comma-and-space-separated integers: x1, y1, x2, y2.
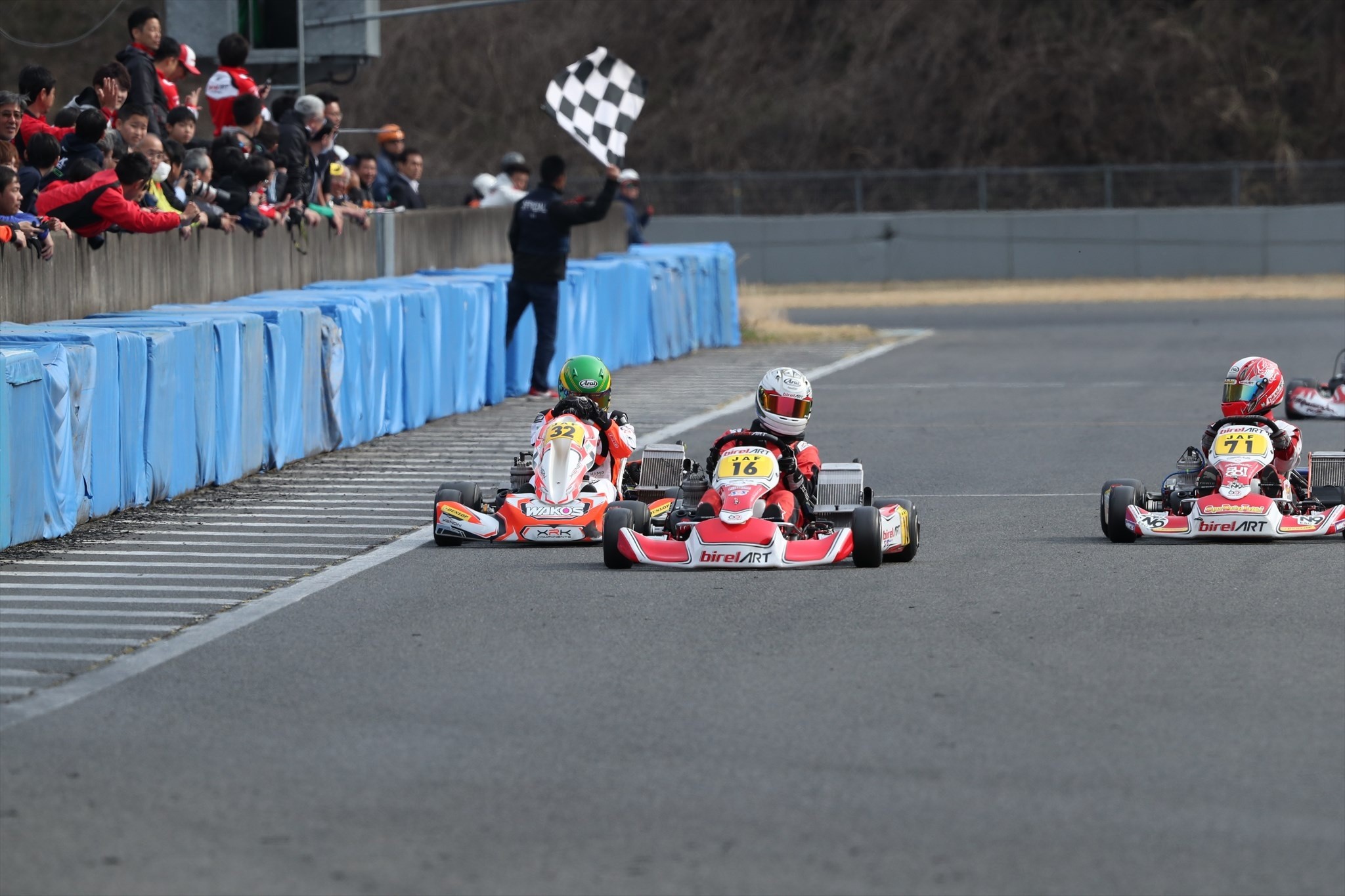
557, 355, 612, 411
376, 124, 405, 143
617, 168, 640, 184
1220, 356, 1284, 417
755, 367, 814, 436
498, 151, 526, 174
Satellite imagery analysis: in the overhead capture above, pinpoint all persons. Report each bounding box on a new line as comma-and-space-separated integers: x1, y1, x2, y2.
614, 168, 654, 241
505, 153, 621, 400
516, 355, 637, 504
0, 7, 531, 262
695, 367, 821, 537
1196, 356, 1302, 515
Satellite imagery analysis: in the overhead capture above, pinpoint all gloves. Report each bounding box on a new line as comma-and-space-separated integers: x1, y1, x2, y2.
777, 444, 798, 475
705, 447, 721, 472
552, 395, 608, 427
1201, 426, 1217, 451
27, 221, 48, 257
1269, 429, 1291, 451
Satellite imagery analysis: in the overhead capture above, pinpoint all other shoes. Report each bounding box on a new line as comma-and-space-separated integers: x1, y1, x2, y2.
580, 485, 597, 493
694, 502, 715, 522
518, 483, 535, 493
1197, 465, 1218, 498
1260, 464, 1283, 499
526, 386, 557, 400
761, 504, 783, 530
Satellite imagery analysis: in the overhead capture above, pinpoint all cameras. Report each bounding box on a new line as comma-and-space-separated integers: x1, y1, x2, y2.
181, 169, 231, 206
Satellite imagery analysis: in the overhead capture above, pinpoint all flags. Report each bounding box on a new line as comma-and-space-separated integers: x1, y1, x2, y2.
542, 45, 646, 171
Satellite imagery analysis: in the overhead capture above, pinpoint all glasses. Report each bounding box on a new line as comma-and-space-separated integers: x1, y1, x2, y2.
2, 111, 23, 120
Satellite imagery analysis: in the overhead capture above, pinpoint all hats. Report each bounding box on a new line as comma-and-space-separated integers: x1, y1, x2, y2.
295, 95, 325, 116
179, 43, 201, 75
